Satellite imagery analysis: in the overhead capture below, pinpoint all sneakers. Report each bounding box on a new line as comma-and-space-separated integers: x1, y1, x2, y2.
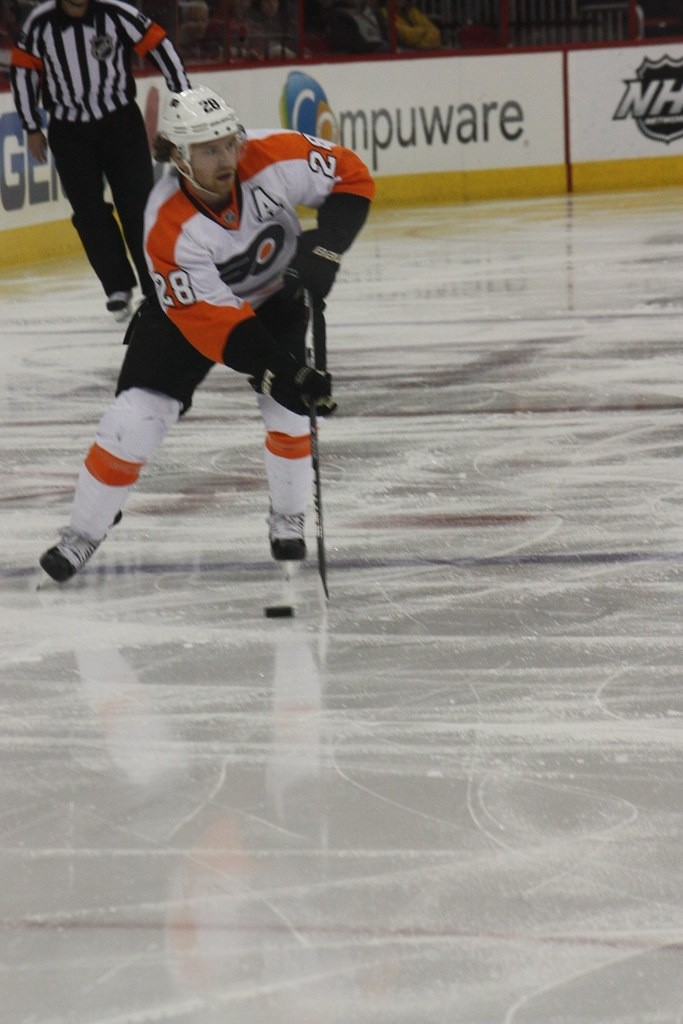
106, 292, 133, 311
40, 526, 107, 583
268, 509, 308, 559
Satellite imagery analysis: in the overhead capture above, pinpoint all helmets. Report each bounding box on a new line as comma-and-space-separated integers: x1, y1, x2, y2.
157, 85, 247, 160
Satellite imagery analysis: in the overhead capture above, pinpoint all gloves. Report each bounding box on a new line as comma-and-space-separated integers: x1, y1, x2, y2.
286, 229, 370, 297
249, 340, 337, 415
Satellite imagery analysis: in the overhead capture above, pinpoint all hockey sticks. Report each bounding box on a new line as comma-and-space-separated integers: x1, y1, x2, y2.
303, 297, 331, 600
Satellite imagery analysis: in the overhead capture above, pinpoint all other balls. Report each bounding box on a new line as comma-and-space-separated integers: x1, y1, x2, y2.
263, 605, 295, 618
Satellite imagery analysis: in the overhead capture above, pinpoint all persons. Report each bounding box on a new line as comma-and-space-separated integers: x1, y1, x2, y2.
36, 83, 378, 593
174, 0, 442, 71
10, 0, 195, 324
